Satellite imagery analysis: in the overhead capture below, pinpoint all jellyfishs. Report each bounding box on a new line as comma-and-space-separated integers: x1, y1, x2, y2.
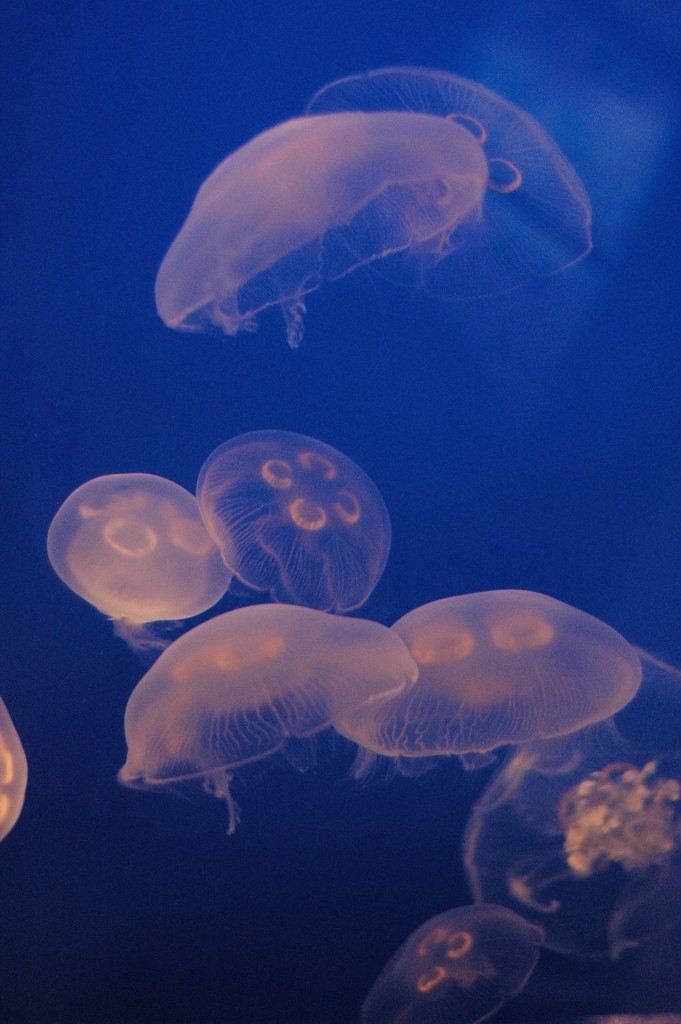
1, 429, 680, 1024
152, 66, 593, 352
